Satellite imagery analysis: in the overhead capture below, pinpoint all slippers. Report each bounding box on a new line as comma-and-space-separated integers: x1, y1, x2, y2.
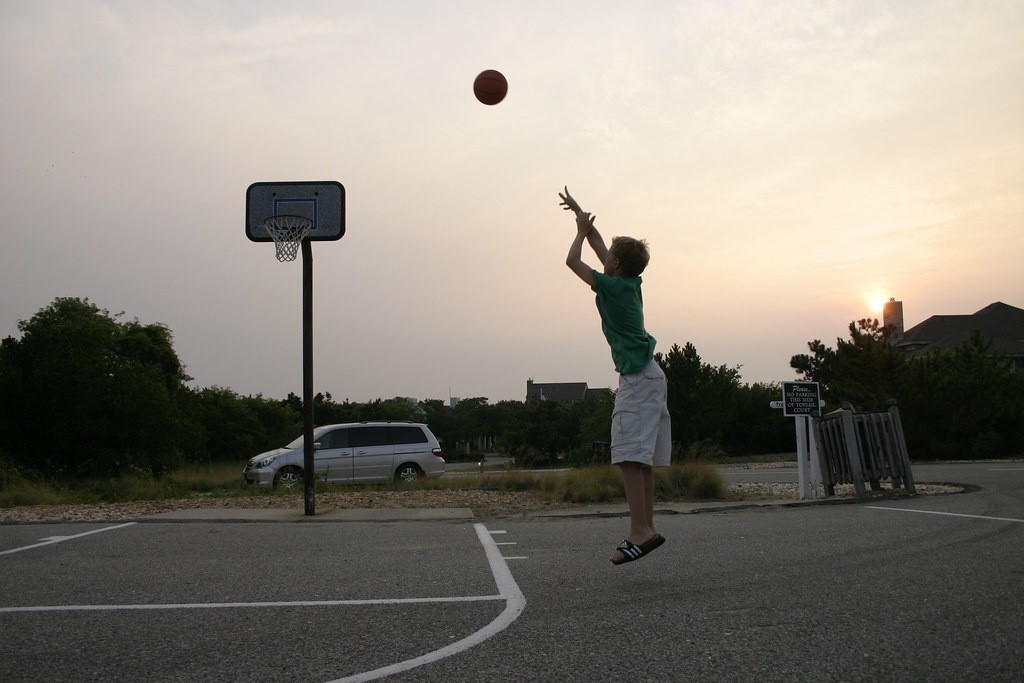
612, 534, 665, 566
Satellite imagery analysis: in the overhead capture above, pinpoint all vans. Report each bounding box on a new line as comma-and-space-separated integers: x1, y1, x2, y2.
243, 419, 447, 493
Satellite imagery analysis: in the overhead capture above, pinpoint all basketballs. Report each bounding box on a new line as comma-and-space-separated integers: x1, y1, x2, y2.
473, 69, 509, 105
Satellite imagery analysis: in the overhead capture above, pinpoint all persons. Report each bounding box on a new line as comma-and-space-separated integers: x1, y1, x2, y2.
557, 185, 672, 565
